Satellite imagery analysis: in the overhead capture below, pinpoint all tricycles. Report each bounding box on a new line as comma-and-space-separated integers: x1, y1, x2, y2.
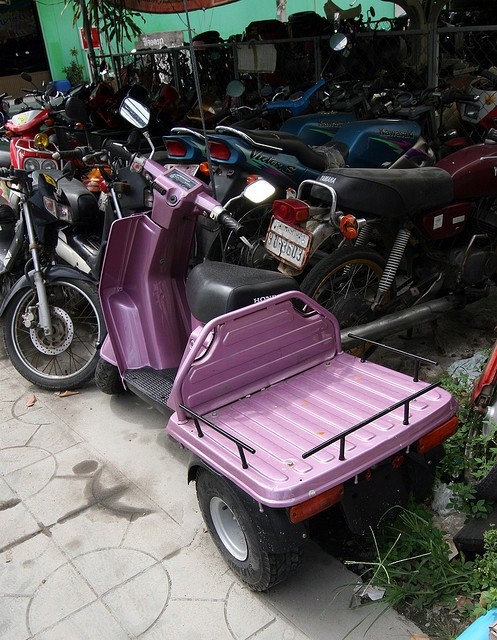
91, 94, 464, 595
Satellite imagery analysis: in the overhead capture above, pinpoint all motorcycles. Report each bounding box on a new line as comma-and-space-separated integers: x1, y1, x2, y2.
0, 159, 107, 394
464, 95, 497, 305
92, 0, 497, 94
0, 70, 91, 168
155, 128, 464, 360
91, 95, 464, 127
24, 131, 155, 285
